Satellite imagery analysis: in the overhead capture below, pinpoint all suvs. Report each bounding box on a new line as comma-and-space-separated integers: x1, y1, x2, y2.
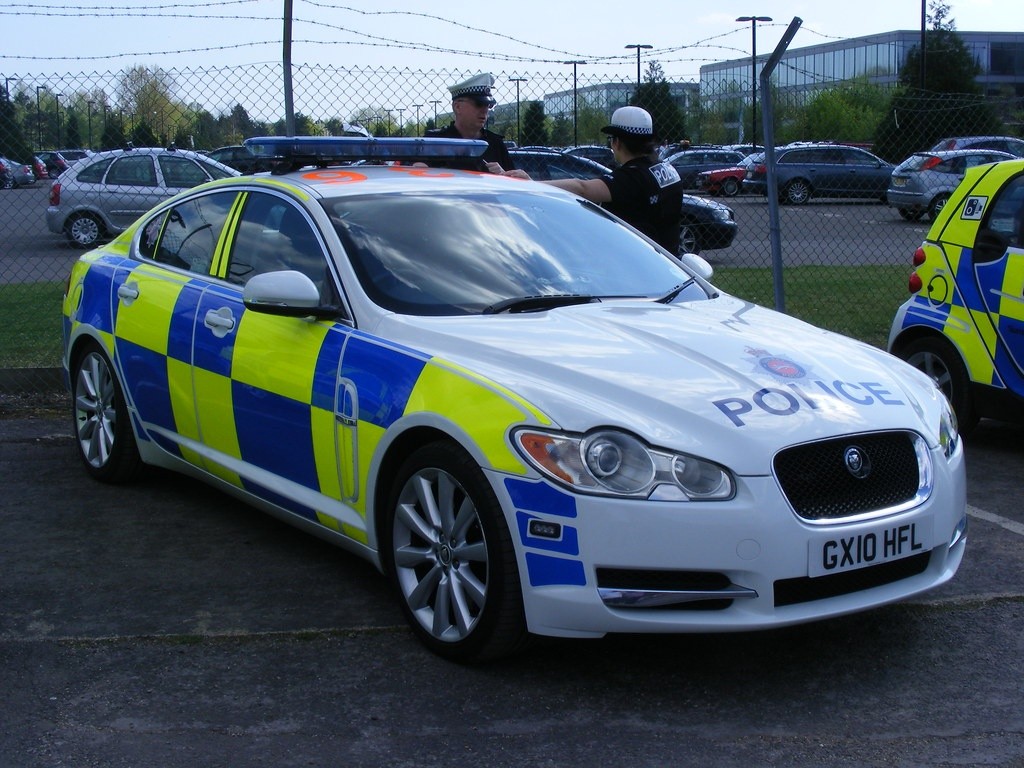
743, 143, 896, 206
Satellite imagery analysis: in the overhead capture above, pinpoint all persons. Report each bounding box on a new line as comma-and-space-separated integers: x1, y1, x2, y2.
677, 140, 692, 151
504, 106, 682, 261
658, 140, 670, 158
401, 73, 515, 177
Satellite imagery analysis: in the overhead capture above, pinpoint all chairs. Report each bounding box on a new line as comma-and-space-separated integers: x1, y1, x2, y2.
276, 207, 356, 304
949, 158, 966, 175
148, 162, 170, 187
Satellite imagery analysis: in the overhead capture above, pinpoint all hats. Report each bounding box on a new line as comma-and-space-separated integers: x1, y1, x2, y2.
601, 106, 653, 140
447, 73, 497, 103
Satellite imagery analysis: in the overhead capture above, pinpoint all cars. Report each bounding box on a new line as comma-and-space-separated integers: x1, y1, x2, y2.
502, 141, 765, 262
885, 160, 1024, 430
887, 149, 1019, 224
931, 136, 1024, 157
0, 146, 244, 249
59, 131, 968, 664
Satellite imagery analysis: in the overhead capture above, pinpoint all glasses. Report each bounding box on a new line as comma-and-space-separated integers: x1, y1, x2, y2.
607, 136, 615, 142
458, 100, 494, 109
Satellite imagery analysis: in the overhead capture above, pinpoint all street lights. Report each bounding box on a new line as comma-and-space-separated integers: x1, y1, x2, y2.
87, 101, 96, 149
103, 105, 176, 146
429, 100, 442, 129
36, 86, 47, 151
509, 78, 528, 149
412, 105, 423, 137
55, 93, 64, 151
6, 78, 17, 146
356, 108, 406, 137
624, 44, 654, 106
563, 60, 587, 148
735, 16, 773, 154
